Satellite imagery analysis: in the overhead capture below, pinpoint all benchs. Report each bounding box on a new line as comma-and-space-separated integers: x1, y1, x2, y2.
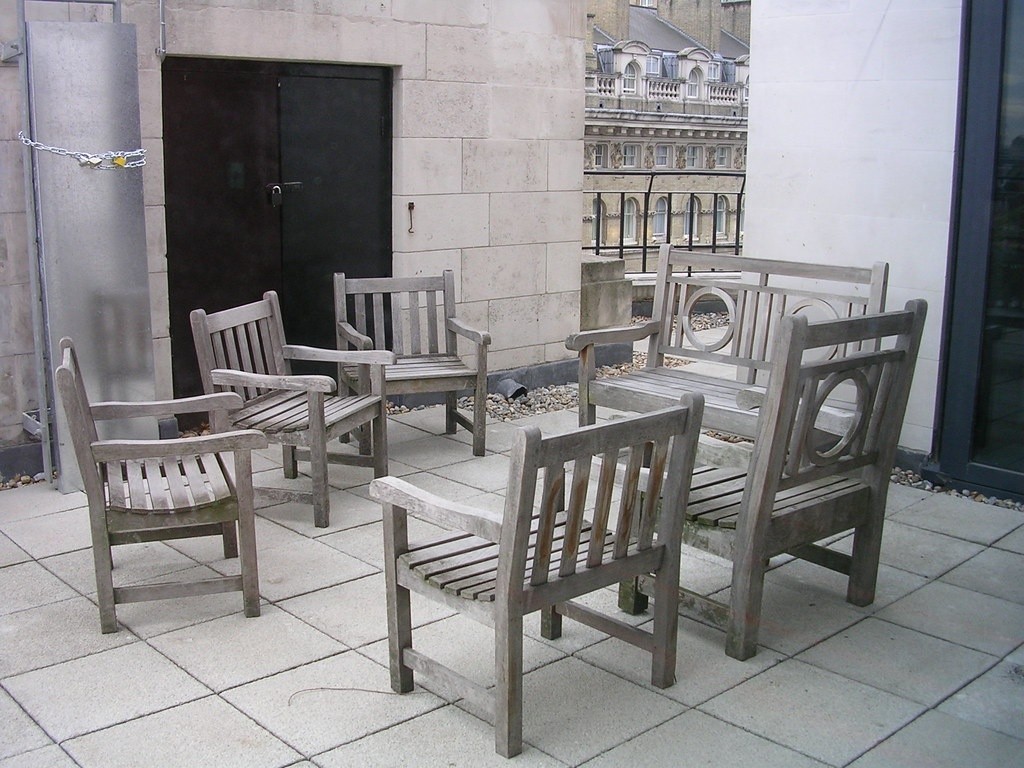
565, 244, 890, 539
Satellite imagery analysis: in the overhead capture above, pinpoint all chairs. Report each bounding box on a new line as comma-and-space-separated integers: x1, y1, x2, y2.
55, 337, 268, 635
368, 391, 706, 760
617, 296, 928, 661
189, 291, 397, 529
332, 269, 492, 457
